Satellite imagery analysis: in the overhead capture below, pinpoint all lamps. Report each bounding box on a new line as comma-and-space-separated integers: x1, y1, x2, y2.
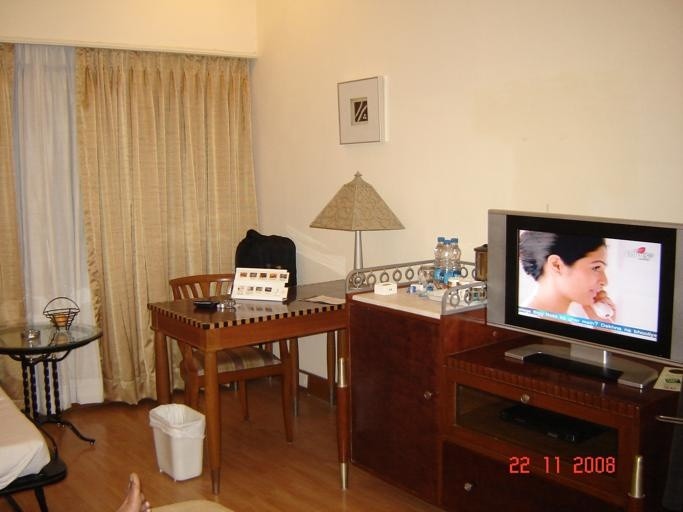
309, 170, 406, 285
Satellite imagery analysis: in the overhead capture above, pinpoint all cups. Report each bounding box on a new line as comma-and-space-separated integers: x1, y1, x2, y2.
222, 298, 236, 309
447, 277, 487, 303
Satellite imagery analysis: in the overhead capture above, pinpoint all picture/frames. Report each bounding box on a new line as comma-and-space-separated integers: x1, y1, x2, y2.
337, 75, 386, 145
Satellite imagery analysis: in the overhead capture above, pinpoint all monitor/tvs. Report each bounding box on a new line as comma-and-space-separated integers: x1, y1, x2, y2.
487, 208, 683, 391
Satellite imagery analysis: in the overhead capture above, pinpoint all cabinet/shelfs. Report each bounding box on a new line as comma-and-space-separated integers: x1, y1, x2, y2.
345, 259, 683, 511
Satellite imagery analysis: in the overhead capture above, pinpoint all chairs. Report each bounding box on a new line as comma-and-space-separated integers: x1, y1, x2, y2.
168, 273, 295, 444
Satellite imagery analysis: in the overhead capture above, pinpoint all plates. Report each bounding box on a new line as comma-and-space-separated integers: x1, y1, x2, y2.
193, 300, 220, 309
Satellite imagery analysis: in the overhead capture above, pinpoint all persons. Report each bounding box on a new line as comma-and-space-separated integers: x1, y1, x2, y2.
517, 227, 616, 329
115, 471, 152, 511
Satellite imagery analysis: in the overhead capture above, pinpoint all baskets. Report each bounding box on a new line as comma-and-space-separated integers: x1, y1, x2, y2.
43, 297, 80, 330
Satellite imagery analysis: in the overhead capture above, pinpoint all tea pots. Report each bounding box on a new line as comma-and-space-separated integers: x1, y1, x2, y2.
472, 244, 488, 280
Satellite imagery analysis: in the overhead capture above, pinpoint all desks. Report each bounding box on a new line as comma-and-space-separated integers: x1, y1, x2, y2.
146, 278, 359, 496
1, 323, 104, 445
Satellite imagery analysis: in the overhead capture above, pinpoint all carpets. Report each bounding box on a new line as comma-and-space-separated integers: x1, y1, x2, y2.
149, 499, 237, 512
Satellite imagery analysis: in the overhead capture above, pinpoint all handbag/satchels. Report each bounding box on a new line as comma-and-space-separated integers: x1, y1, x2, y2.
235, 230, 297, 287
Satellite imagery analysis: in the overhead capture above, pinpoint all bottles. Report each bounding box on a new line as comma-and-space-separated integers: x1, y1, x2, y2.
432, 236, 462, 288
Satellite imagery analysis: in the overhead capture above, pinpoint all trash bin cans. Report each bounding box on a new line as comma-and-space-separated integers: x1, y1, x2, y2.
148, 402, 207, 482
